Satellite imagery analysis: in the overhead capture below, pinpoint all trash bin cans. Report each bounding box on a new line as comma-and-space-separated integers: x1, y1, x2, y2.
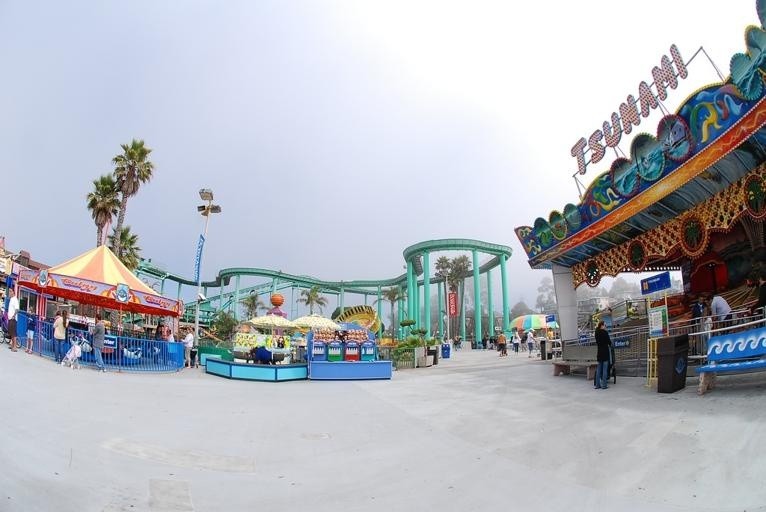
441, 344, 450, 358
541, 340, 552, 360
427, 346, 439, 364
657, 333, 690, 393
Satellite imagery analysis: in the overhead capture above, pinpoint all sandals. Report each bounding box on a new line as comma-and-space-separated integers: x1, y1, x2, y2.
8, 347, 17, 352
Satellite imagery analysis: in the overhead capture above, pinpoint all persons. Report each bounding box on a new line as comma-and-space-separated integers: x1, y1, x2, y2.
577, 321, 620, 343
444, 327, 561, 358
690, 293, 709, 356
595, 320, 612, 389
745, 277, 759, 289
749, 272, 766, 327
0, 287, 200, 373
706, 294, 733, 335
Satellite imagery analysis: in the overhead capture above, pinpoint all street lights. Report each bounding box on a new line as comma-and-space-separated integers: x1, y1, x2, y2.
193, 188, 220, 357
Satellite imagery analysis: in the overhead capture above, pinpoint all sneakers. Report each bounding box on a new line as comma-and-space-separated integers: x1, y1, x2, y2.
596, 384, 608, 389
25, 349, 32, 354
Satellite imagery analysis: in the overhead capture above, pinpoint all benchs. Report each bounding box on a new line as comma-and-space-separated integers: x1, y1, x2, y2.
694, 326, 766, 395
553, 346, 598, 381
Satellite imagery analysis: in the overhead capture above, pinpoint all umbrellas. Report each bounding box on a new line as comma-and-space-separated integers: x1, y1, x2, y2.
291, 313, 342, 360
240, 312, 301, 360
